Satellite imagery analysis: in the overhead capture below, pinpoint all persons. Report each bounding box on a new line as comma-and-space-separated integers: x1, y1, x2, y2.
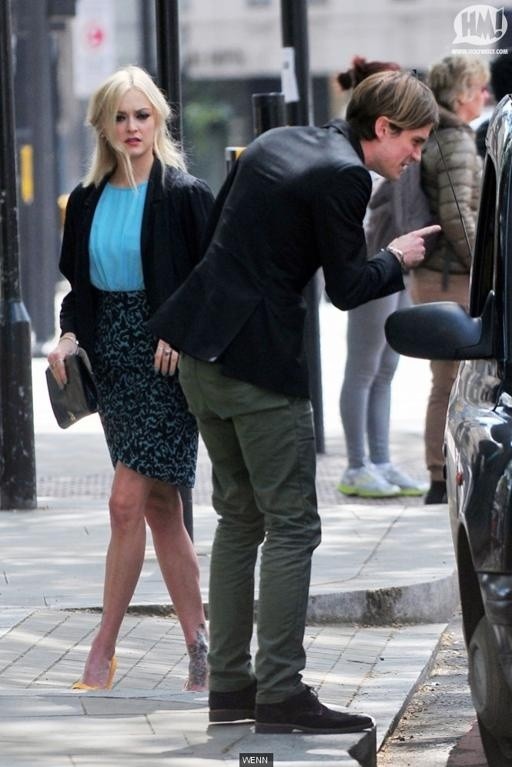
45, 62, 220, 699
141, 64, 452, 737
405, 46, 495, 503
333, 51, 440, 502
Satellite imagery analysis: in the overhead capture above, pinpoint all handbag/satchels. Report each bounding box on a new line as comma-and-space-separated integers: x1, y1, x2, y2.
45, 355, 98, 429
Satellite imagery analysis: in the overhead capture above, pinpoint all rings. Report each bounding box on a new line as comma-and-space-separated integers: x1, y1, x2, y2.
48, 361, 58, 369
164, 347, 174, 358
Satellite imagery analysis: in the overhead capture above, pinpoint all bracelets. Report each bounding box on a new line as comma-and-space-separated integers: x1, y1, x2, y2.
57, 335, 79, 347
385, 244, 407, 271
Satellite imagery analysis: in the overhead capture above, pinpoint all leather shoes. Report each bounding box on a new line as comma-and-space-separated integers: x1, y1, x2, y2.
209, 678, 257, 722
254, 684, 374, 734
425, 480, 448, 503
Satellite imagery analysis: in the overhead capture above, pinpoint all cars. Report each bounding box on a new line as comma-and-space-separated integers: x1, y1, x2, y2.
383, 90, 510, 763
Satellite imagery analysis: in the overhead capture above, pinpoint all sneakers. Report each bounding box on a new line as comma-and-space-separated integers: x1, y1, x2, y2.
383, 464, 424, 496
338, 464, 401, 498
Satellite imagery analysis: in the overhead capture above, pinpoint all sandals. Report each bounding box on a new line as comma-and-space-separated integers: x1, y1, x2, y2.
71, 656, 117, 691
